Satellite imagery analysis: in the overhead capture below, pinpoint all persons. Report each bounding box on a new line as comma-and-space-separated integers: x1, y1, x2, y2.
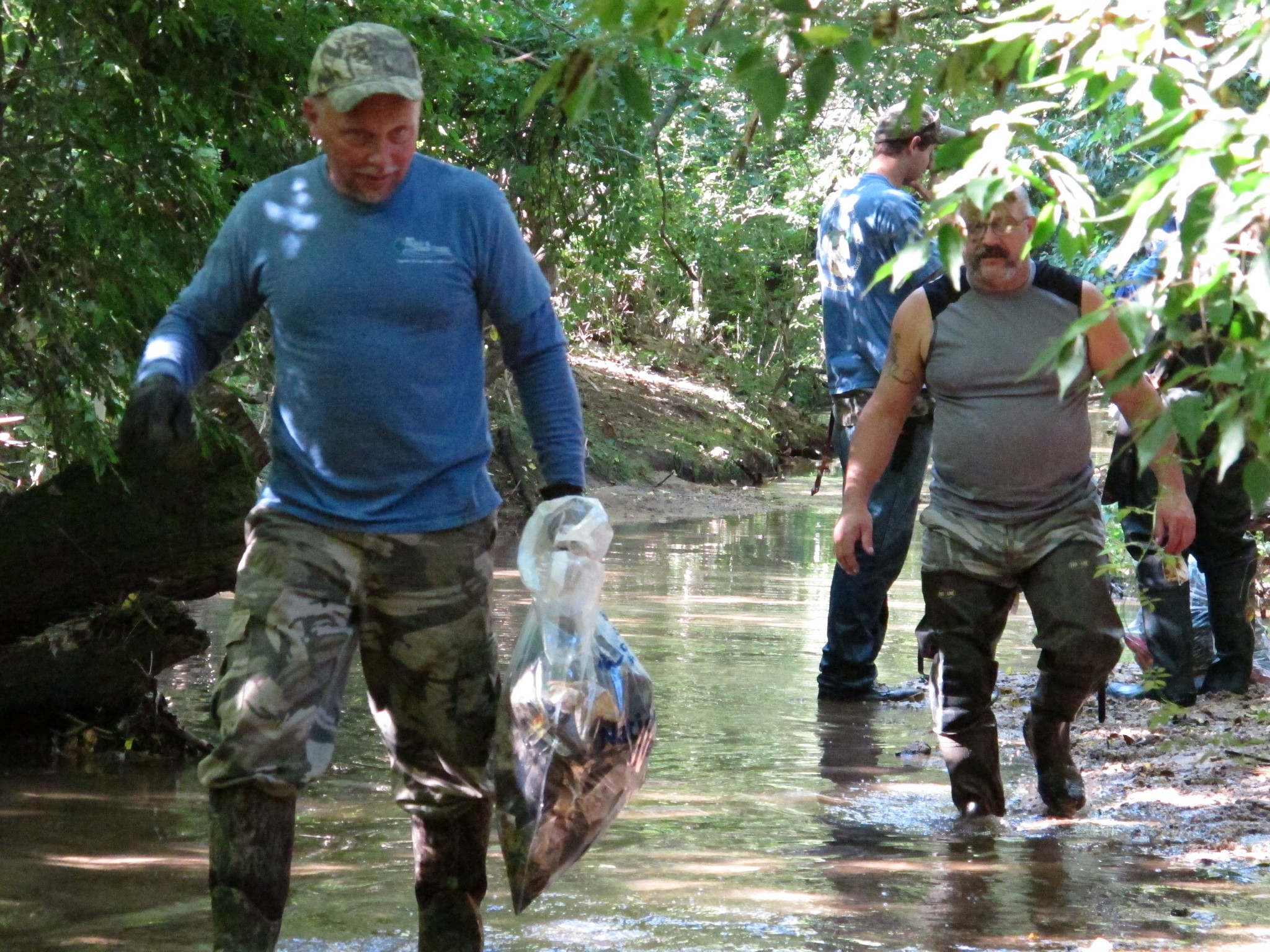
816, 100, 1255, 817
121, 22, 590, 952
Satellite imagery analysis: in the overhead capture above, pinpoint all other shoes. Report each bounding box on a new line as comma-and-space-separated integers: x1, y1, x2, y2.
855, 684, 922, 701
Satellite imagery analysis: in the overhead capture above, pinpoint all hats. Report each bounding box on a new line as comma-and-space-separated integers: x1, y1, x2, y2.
306, 22, 424, 110
876, 100, 965, 145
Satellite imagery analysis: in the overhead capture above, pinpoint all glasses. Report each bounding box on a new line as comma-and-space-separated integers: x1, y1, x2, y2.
961, 217, 1025, 241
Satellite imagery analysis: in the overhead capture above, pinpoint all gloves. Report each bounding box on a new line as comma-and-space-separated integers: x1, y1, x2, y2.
119, 376, 195, 461
539, 481, 581, 536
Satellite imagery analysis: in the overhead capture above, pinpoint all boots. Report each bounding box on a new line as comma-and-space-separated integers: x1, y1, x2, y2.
209, 786, 297, 952
915, 569, 1016, 816
1194, 556, 1257, 692
1106, 580, 1199, 707
1023, 541, 1125, 812
412, 796, 492, 952
